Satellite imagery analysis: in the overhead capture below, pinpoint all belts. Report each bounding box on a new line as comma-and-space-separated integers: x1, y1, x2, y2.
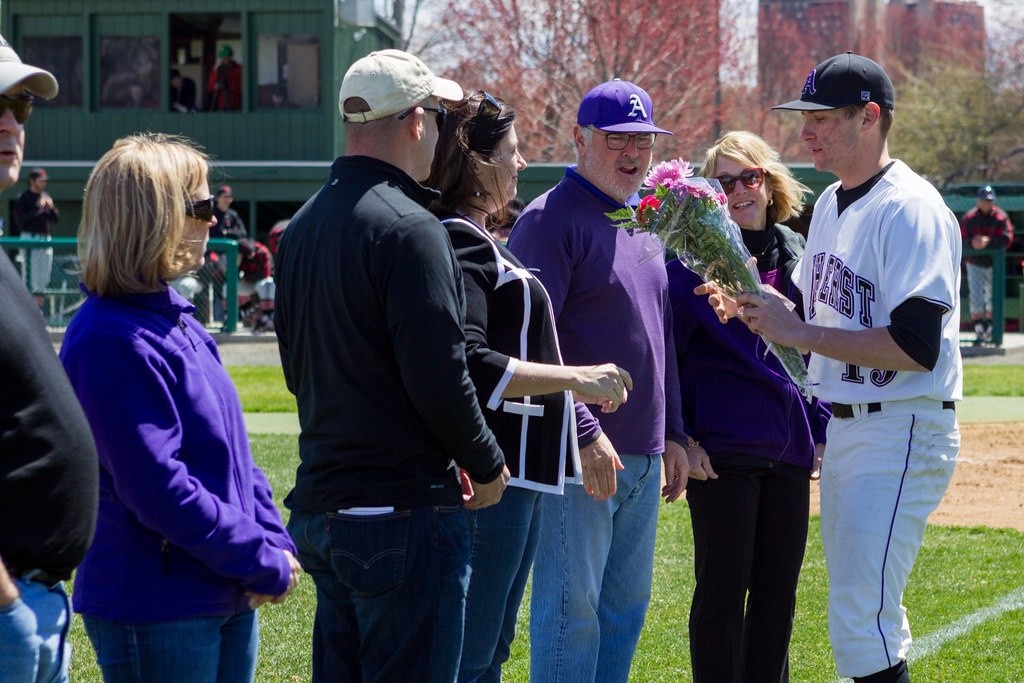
829, 398, 960, 422
4, 554, 67, 593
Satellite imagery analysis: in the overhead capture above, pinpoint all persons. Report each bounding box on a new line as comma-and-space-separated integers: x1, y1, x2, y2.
14, 168, 60, 293
237, 239, 274, 334
661, 129, 833, 683
0, 34, 101, 683
504, 78, 690, 683
170, 68, 196, 113
694, 50, 964, 683
209, 184, 246, 321
418, 90, 634, 683
958, 185, 1014, 347
272, 49, 511, 683
58, 133, 301, 683
207, 46, 242, 111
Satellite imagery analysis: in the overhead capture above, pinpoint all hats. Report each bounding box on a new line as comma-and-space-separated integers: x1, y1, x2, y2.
217, 184, 234, 197
977, 185, 997, 202
29, 169, 50, 182
576, 77, 674, 136
219, 46, 233, 58
1, 31, 60, 101
770, 51, 897, 114
337, 48, 464, 124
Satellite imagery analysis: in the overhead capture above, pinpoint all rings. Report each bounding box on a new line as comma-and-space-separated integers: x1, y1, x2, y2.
299, 574, 300, 577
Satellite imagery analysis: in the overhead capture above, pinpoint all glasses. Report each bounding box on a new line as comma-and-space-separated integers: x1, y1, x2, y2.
397, 105, 449, 136
710, 166, 769, 196
183, 194, 220, 223
1, 93, 35, 125
583, 125, 657, 149
457, 89, 503, 148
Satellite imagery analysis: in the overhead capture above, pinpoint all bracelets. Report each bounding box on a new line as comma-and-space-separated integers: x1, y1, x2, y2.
814, 329, 825, 350
687, 441, 700, 450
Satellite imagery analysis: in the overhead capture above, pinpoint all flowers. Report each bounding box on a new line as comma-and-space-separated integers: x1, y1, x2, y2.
605, 159, 813, 401
